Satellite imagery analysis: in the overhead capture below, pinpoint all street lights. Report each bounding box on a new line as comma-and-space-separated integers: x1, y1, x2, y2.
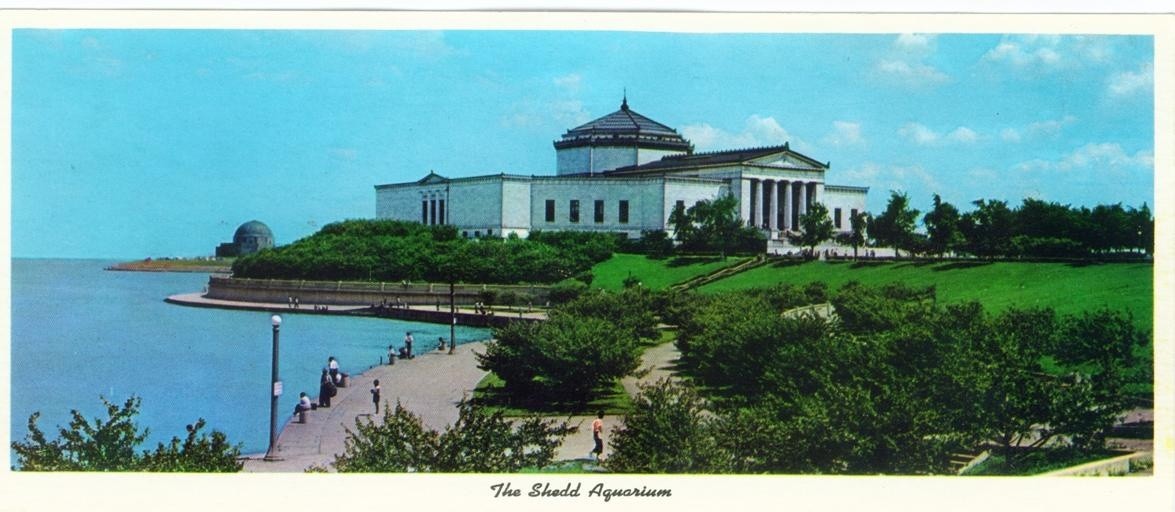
266, 313, 286, 461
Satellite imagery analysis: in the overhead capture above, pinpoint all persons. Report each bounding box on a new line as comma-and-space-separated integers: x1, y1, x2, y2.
293, 391, 312, 416
528, 301, 532, 312
314, 303, 328, 313
774, 246, 877, 259
388, 331, 413, 364
436, 297, 441, 311
473, 300, 484, 314
316, 367, 332, 408
288, 295, 293, 307
328, 355, 343, 387
396, 295, 401, 308
294, 296, 299, 308
370, 379, 380, 413
591, 411, 605, 462
438, 337, 447, 350
